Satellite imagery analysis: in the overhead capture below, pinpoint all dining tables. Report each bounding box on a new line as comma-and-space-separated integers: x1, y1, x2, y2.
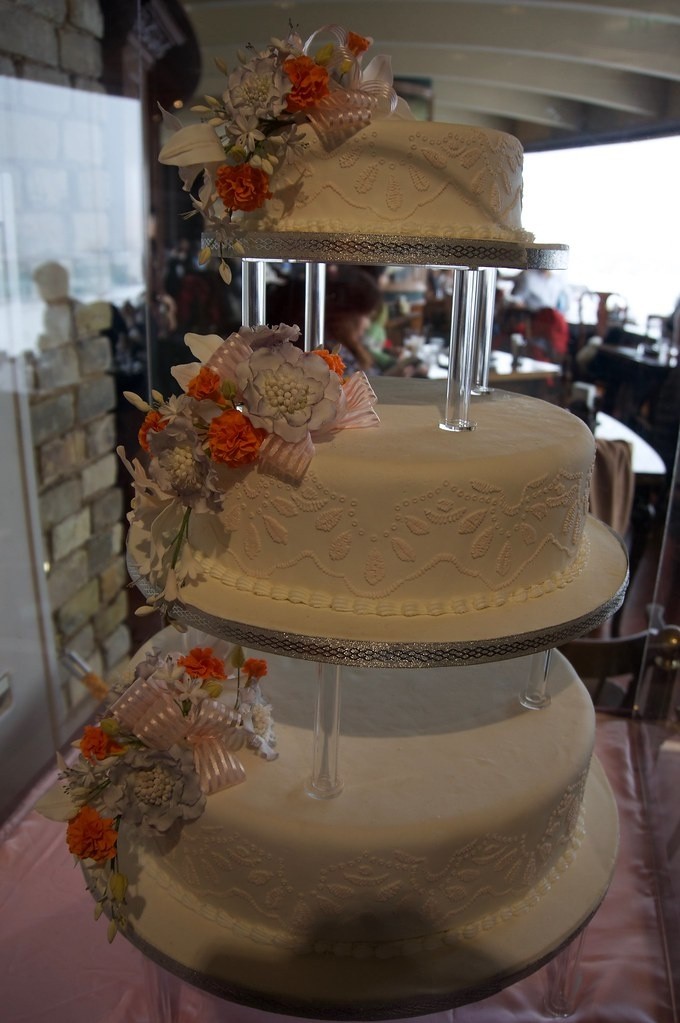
375, 269, 680, 479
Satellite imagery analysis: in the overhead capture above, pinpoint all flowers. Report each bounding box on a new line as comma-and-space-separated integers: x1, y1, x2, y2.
112, 321, 367, 636
154, 21, 418, 290
28, 639, 281, 942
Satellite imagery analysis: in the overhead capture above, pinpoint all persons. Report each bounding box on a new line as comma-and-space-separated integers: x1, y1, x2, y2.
146, 235, 571, 406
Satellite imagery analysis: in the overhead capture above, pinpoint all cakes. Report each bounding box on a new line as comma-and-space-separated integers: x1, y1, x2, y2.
38, 22, 625, 1006
